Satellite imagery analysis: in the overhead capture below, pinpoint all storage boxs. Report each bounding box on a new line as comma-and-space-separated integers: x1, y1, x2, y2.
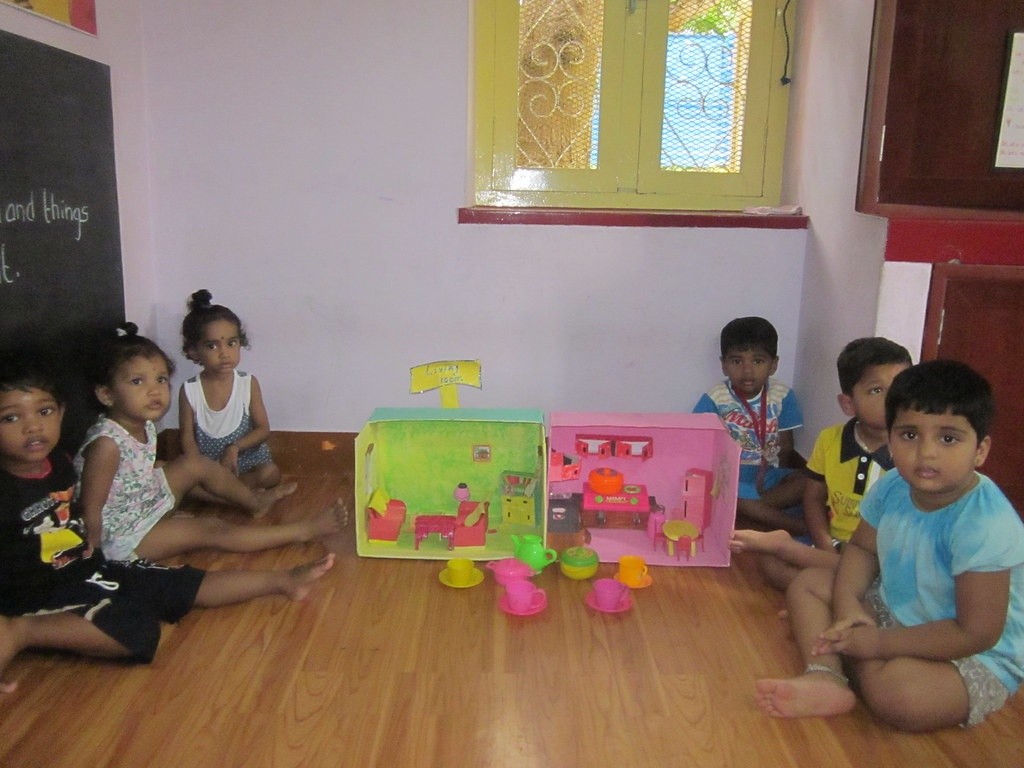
545, 410, 739, 568
354, 407, 548, 564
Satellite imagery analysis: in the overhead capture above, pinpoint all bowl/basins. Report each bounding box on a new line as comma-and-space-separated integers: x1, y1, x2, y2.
486, 558, 536, 584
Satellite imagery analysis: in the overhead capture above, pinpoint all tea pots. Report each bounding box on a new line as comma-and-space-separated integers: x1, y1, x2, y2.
511, 534, 558, 574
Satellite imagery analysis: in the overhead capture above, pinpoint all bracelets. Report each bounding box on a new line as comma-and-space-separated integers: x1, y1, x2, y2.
226, 442, 240, 451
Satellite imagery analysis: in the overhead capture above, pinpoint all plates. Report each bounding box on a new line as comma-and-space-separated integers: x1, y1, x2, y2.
586, 592, 634, 612
499, 590, 548, 616
439, 568, 484, 589
613, 572, 653, 589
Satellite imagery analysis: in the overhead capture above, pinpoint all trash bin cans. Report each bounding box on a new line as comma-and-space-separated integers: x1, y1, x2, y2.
549, 490, 583, 533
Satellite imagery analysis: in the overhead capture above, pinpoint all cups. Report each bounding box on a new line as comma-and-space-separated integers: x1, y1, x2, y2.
620, 555, 648, 586
448, 558, 473, 586
505, 580, 546, 612
594, 578, 630, 609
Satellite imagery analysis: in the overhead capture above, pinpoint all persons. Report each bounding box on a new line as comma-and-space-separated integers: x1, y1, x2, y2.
0, 364, 334, 694
179, 290, 280, 507
692, 317, 807, 538
728, 338, 913, 642
72, 323, 347, 561
753, 360, 1024, 730
